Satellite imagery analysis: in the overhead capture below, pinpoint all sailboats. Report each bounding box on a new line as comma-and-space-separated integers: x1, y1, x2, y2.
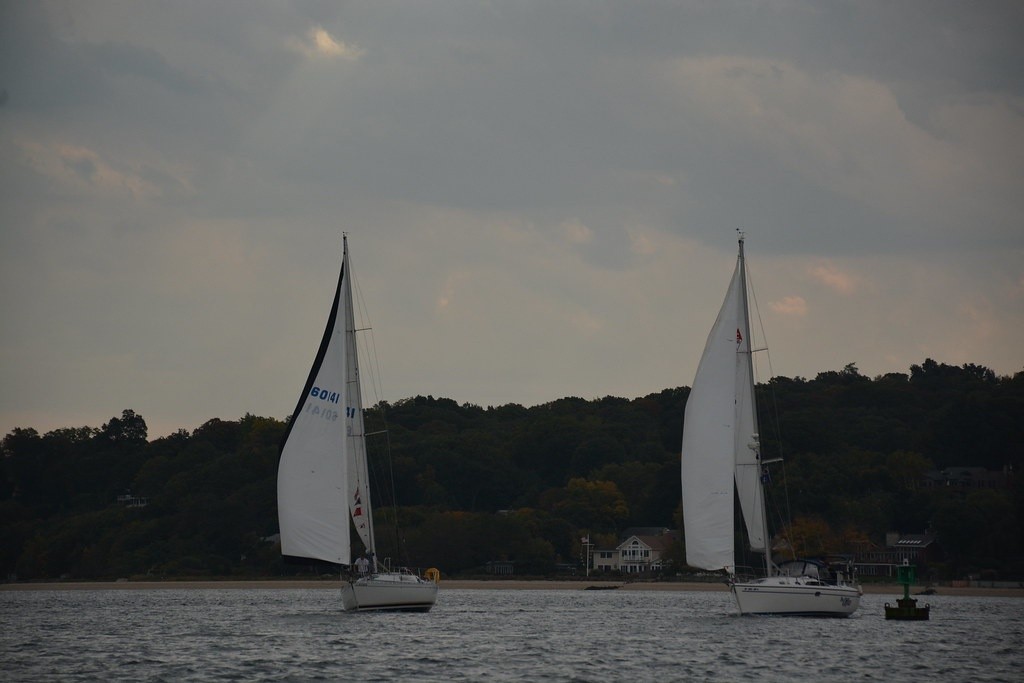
681, 227, 864, 616
276, 231, 439, 615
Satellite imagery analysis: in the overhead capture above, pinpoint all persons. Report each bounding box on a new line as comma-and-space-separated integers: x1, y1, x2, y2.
354, 553, 369, 577
